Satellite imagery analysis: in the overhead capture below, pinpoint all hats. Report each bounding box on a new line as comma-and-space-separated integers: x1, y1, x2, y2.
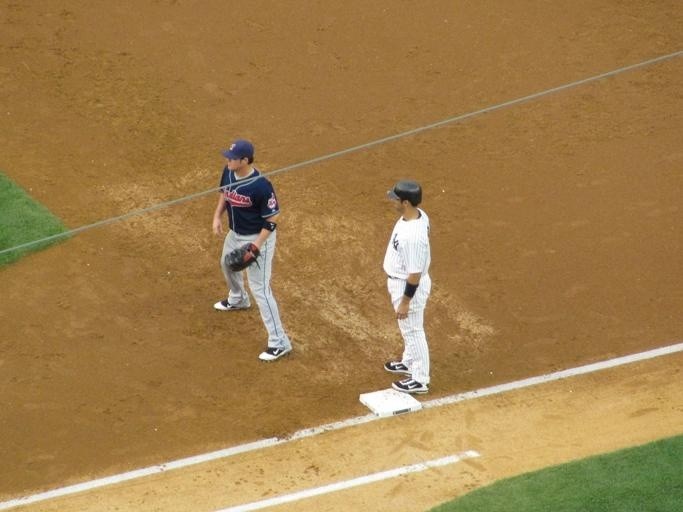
223, 140, 254, 159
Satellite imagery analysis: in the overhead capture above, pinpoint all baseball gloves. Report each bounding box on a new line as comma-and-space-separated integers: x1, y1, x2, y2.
225, 241, 262, 273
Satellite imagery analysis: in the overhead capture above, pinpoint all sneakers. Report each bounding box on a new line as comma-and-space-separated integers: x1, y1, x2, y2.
384, 359, 412, 377
213, 298, 251, 311
258, 344, 292, 363
392, 378, 430, 395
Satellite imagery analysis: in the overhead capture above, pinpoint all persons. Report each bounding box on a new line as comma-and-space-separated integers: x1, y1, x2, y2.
383, 180, 432, 394
212, 140, 293, 362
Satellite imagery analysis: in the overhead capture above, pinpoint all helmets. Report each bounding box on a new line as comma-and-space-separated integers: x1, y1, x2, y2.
386, 181, 422, 205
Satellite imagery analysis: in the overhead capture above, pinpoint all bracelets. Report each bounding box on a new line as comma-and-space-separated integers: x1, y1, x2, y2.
404, 282, 418, 299
